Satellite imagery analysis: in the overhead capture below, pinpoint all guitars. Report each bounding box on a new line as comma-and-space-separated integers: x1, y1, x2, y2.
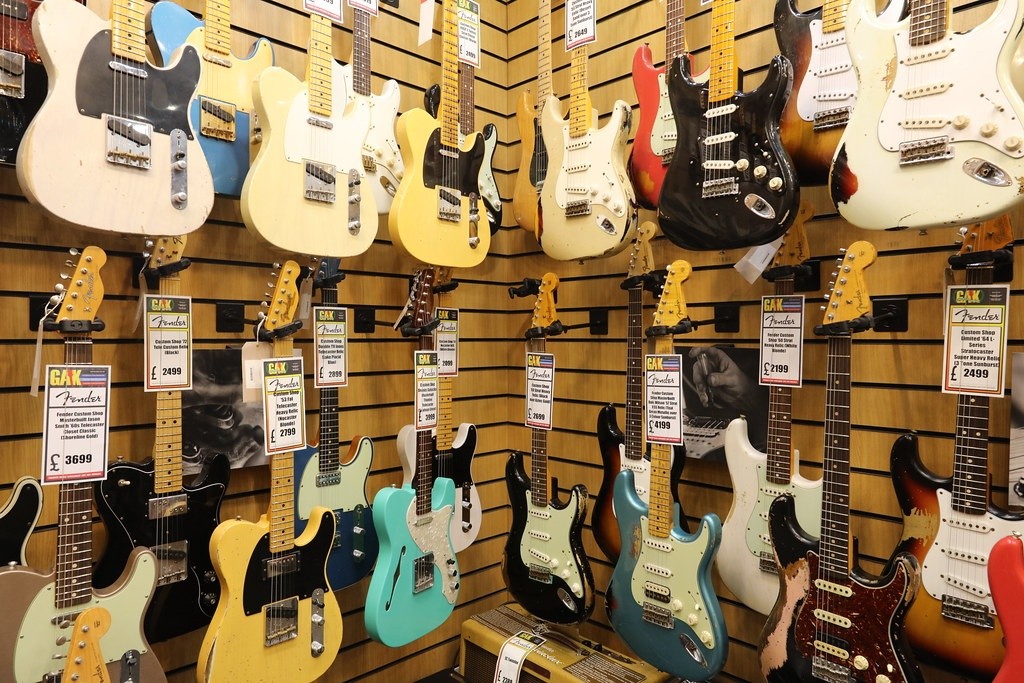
0, 199, 1024, 683
0, 0, 1024, 269
